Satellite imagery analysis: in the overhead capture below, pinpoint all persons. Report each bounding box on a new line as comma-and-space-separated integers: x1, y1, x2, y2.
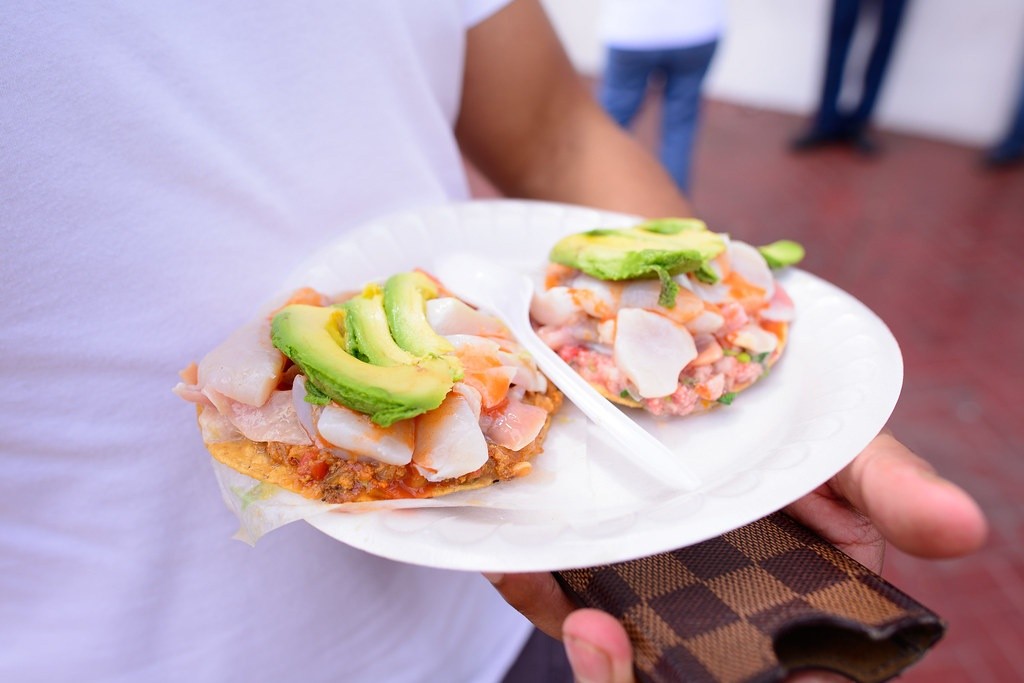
595, 0, 726, 195
0, 0, 987, 683
788, 0, 909, 154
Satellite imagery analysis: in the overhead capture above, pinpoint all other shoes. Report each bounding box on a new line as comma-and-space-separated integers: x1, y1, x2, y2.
797, 120, 841, 149
841, 120, 872, 153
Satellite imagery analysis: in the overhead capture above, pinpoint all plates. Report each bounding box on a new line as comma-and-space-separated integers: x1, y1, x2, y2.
250, 198, 903, 572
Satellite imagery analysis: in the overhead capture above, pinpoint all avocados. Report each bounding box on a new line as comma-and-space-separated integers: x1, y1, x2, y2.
272, 270, 466, 429
547, 217, 727, 307
756, 240, 805, 267
